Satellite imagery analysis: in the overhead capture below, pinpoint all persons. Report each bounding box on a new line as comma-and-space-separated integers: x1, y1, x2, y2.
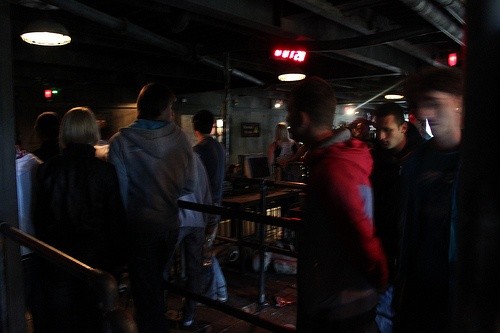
370, 103, 426, 333
15, 82, 308, 333
285, 77, 389, 333
382, 68, 500, 333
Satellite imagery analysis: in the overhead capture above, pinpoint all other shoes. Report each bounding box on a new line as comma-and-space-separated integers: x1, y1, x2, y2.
205, 299, 227, 308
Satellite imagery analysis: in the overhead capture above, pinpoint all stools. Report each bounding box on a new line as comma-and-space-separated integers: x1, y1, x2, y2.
289, 207, 303, 218
280, 218, 301, 248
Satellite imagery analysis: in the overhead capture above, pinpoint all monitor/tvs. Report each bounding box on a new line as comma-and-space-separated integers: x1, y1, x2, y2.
238, 153, 270, 179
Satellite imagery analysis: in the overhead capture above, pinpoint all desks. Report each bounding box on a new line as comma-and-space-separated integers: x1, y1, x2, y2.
221, 190, 291, 246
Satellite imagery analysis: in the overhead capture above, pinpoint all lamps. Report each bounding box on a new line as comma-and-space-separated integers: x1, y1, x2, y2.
18, 11, 71, 47
